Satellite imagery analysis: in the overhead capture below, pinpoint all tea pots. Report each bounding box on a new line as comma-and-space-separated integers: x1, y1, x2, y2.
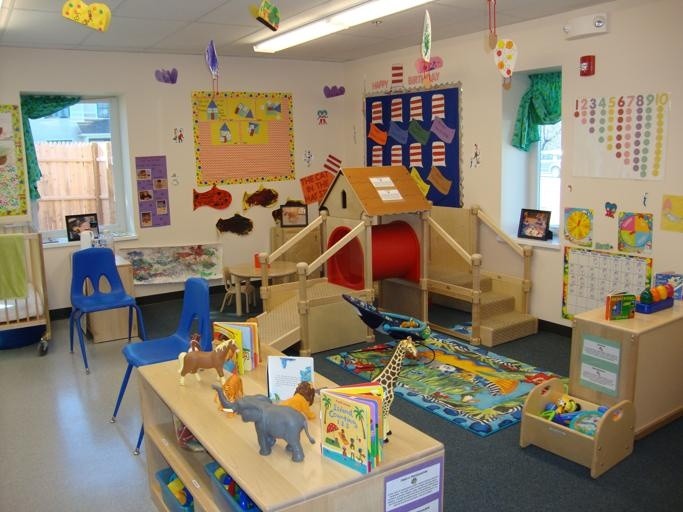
77, 229, 93, 250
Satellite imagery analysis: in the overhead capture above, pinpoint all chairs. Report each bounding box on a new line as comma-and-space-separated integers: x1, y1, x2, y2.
220, 265, 257, 314
104, 276, 212, 458
66, 245, 148, 376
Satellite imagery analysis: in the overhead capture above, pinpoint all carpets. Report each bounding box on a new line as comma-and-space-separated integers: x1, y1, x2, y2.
325, 322, 566, 437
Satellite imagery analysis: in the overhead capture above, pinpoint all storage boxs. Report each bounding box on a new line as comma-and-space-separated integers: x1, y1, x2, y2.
519, 377, 637, 478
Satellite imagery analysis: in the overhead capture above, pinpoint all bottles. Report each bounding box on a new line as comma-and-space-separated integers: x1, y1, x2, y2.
92, 233, 106, 248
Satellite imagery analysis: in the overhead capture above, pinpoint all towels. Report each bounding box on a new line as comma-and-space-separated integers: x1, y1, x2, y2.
0, 233, 28, 300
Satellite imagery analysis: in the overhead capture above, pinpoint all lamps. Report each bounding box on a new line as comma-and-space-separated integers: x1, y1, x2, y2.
252, 0, 430, 53
563, 11, 607, 41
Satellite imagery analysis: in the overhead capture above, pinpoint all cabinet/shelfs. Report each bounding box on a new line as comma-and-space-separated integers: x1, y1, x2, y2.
570, 296, 682, 447
269, 227, 321, 283
70, 251, 139, 345
137, 340, 446, 512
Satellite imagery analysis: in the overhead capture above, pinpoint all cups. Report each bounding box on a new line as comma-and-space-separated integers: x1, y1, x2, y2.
253, 252, 260, 268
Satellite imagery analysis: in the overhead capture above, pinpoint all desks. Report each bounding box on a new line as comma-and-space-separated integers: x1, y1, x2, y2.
228, 261, 301, 316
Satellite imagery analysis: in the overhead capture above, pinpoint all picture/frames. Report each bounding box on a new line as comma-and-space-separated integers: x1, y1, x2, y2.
280, 204, 308, 227
65, 213, 101, 242
517, 208, 553, 242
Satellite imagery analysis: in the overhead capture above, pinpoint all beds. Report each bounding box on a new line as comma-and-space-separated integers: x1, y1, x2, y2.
0, 219, 52, 355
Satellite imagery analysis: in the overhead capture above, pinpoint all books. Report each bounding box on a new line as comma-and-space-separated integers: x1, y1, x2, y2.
320, 380, 384, 476
605, 288, 636, 320
213, 320, 260, 374
267, 354, 315, 400
654, 271, 683, 300
